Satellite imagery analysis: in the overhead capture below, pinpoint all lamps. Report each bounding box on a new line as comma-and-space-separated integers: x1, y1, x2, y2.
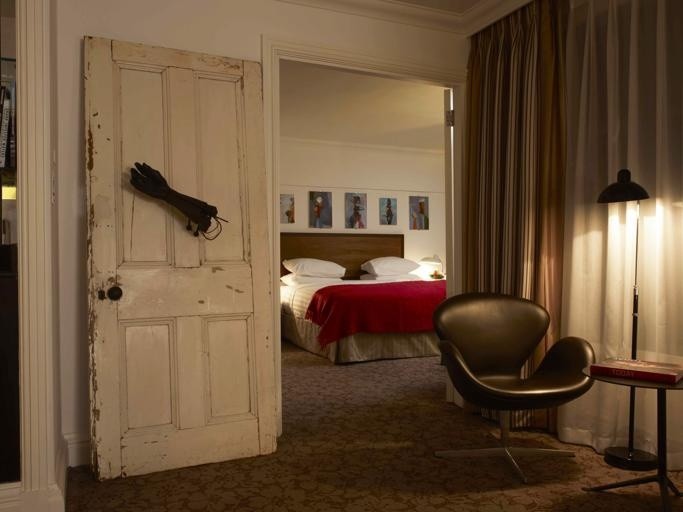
597, 169, 659, 472
420, 255, 445, 280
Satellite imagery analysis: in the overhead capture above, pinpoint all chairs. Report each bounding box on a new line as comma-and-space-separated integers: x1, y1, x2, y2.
433, 293, 595, 484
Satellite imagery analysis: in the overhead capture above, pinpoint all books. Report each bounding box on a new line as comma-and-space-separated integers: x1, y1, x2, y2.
590, 358, 683, 384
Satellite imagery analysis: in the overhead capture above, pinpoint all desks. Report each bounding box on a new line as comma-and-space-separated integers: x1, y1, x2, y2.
582, 363, 682, 511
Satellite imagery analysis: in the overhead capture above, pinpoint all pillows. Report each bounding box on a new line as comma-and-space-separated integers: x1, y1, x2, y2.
280, 273, 343, 286
360, 274, 420, 280
361, 256, 421, 275
282, 258, 346, 277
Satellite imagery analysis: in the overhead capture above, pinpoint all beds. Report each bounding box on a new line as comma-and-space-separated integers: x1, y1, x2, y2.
280, 232, 446, 364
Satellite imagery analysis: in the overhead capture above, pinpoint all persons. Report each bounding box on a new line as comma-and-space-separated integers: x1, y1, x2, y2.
385, 198, 393, 224
414, 198, 429, 230
353, 196, 365, 228
314, 196, 323, 228
